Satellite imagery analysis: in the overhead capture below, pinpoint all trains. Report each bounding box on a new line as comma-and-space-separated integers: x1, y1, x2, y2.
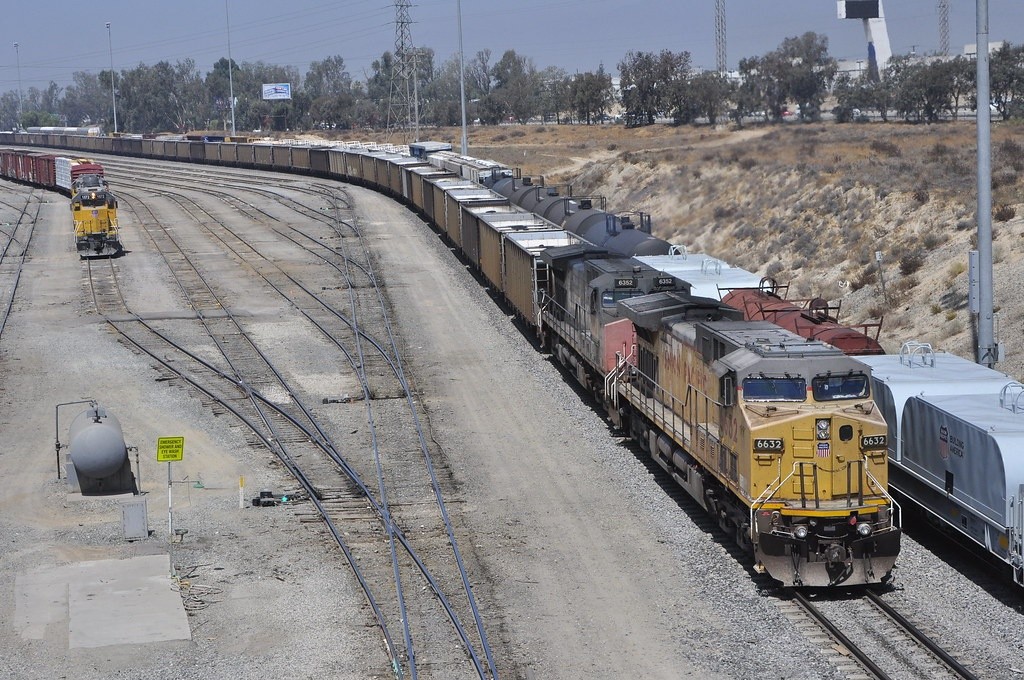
0, 131, 901, 588
0, 149, 123, 259
26, 126, 1024, 588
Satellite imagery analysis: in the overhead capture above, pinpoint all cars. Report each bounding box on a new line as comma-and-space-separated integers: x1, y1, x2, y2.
727, 103, 800, 117
595, 113, 614, 120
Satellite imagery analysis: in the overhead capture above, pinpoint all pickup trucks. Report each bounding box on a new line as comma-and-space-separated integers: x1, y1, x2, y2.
616, 112, 634, 120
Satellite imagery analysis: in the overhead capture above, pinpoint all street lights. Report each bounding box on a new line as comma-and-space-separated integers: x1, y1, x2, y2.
106, 22, 117, 132
857, 61, 864, 76
14, 42, 23, 114
